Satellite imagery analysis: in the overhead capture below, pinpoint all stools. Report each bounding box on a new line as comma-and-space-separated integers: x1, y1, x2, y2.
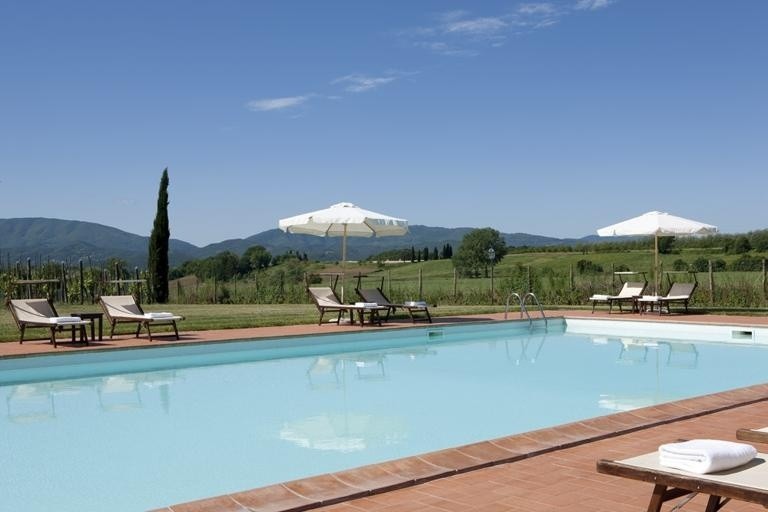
735, 425, 768, 445
596, 436, 768, 512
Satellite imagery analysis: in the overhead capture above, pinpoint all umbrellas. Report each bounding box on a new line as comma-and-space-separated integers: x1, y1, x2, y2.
600, 343, 663, 411
596, 209, 719, 293
278, 202, 409, 304
278, 349, 367, 451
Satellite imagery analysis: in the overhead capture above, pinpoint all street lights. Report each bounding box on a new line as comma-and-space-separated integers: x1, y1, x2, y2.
489, 247, 496, 305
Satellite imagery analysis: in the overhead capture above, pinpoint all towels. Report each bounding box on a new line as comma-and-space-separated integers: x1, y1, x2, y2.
353, 300, 379, 308
641, 294, 661, 300
143, 310, 174, 319
46, 313, 83, 324
592, 293, 612, 299
404, 300, 429, 307
656, 437, 760, 477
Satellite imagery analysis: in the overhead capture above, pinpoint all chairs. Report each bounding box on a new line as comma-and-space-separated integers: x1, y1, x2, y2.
633, 280, 699, 317
587, 279, 649, 315
305, 284, 390, 328
351, 286, 433, 327
303, 348, 390, 381
6, 296, 95, 350
6, 365, 175, 419
95, 294, 187, 343
587, 332, 701, 373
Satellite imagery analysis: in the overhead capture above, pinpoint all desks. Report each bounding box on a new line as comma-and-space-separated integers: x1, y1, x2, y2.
630, 294, 666, 313
69, 311, 105, 342
352, 302, 390, 328
